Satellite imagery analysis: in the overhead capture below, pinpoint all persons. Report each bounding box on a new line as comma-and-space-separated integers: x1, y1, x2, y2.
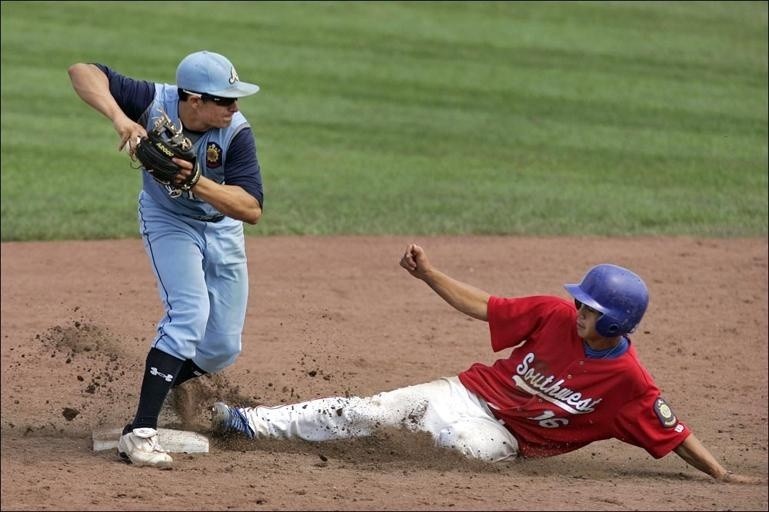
65, 50, 266, 471
203, 242, 768, 489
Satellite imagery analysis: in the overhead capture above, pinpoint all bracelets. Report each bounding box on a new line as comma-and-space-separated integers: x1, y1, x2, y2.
722, 468, 733, 483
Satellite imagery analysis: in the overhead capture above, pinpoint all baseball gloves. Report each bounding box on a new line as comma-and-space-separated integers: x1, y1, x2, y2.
134, 127, 201, 193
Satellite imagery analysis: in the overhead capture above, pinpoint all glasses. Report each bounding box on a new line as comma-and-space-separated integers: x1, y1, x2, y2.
182, 90, 238, 106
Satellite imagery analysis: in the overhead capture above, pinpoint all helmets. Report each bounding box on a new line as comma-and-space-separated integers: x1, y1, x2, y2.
563, 264, 649, 337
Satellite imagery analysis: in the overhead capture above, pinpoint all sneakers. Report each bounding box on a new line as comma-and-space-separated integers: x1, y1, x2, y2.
213, 402, 254, 443
117, 427, 173, 470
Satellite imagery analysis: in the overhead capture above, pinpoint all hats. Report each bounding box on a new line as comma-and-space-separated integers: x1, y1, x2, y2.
175, 51, 260, 99
